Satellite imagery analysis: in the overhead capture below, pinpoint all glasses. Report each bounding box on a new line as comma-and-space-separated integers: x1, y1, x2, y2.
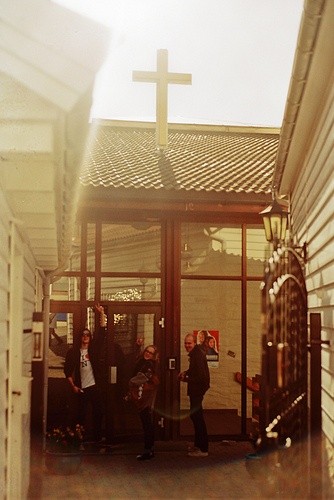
81, 332, 90, 336
146, 349, 154, 355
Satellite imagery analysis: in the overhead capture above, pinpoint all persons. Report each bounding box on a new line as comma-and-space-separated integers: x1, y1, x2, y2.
197, 329, 219, 362
246, 438, 278, 459
64, 301, 112, 454
177, 333, 210, 457
49, 313, 63, 346
123, 336, 169, 460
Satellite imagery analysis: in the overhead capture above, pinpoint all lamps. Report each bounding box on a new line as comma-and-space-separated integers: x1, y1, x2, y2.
258, 186, 308, 262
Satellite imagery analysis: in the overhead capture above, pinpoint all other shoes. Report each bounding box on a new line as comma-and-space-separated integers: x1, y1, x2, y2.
137, 452, 155, 460
188, 448, 208, 456
